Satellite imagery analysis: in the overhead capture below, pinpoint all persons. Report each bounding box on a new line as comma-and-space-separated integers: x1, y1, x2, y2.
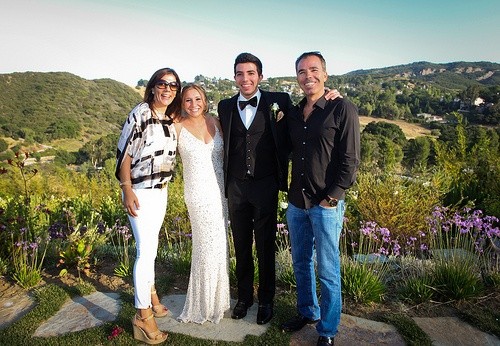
115, 67, 183, 345
280, 51, 360, 346
218, 52, 344, 325
173, 82, 230, 324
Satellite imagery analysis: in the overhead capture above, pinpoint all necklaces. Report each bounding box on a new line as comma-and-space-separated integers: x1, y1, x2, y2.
192, 121, 204, 135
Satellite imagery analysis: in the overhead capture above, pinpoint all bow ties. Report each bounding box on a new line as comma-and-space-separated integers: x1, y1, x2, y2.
238, 96, 258, 111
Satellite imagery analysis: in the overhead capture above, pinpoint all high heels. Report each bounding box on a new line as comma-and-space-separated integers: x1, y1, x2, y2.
151, 289, 170, 319
131, 312, 169, 345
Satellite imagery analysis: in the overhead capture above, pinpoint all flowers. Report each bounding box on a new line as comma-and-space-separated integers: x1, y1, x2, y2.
271, 102, 280, 118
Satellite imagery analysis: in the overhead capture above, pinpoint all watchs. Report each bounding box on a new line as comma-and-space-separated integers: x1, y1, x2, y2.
324, 195, 338, 207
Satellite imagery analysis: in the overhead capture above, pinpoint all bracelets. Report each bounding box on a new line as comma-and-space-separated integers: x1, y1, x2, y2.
119, 181, 133, 188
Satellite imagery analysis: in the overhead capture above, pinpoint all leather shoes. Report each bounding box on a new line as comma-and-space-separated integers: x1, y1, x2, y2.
256, 298, 275, 325
281, 311, 320, 333
316, 335, 336, 346
231, 296, 254, 320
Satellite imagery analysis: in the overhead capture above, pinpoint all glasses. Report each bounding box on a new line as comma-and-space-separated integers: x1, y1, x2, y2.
155, 79, 180, 92
